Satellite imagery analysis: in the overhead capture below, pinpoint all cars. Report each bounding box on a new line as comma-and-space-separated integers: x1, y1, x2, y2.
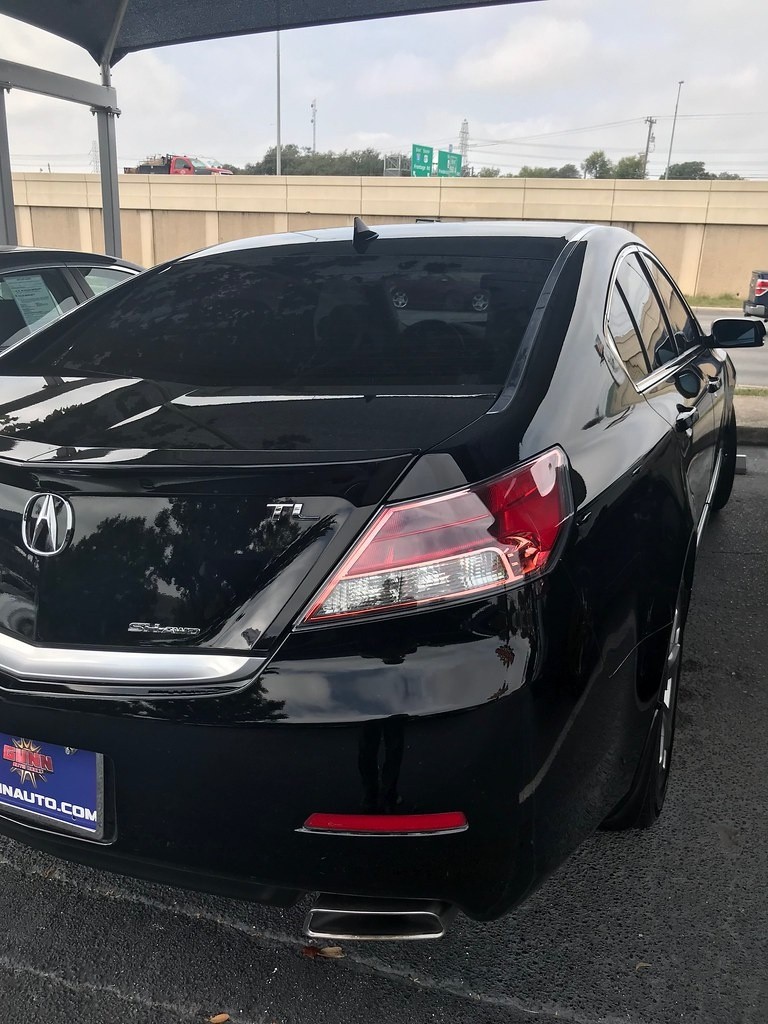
1, 242, 139, 362
6, 210, 768, 922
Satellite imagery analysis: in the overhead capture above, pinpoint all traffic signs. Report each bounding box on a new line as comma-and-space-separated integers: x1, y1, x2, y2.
437, 149, 462, 177
411, 144, 433, 178
